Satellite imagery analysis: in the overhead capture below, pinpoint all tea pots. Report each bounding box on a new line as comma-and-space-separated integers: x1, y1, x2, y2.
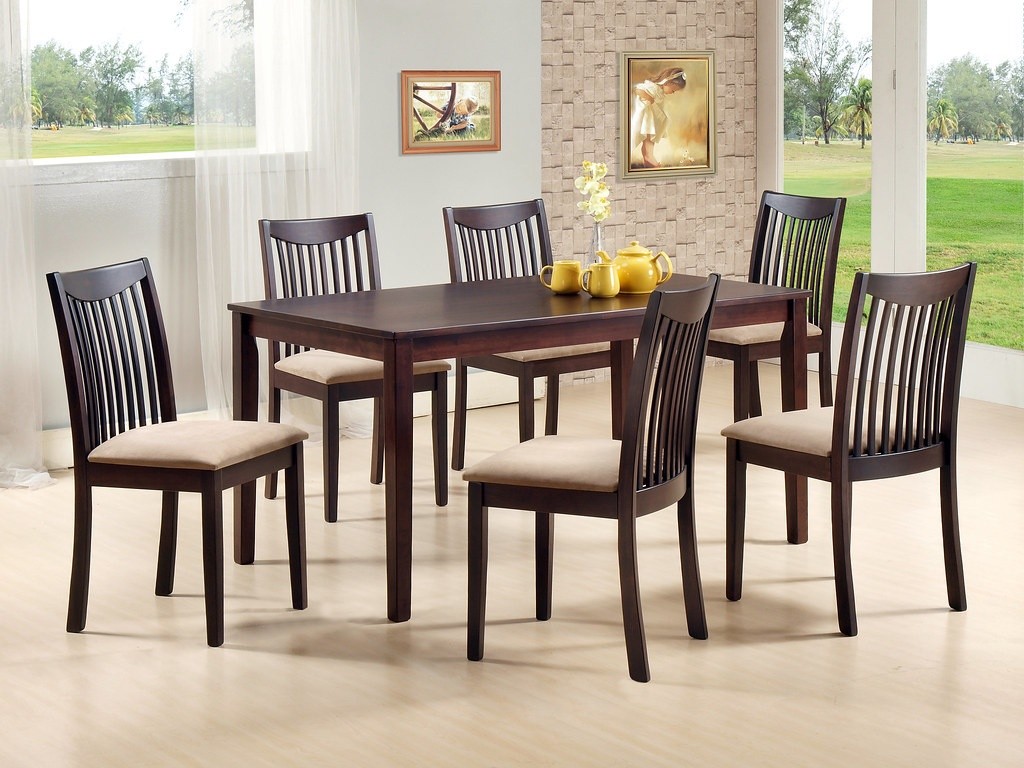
595, 241, 673, 294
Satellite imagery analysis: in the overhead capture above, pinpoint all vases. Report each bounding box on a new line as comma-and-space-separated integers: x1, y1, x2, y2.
588, 224, 608, 266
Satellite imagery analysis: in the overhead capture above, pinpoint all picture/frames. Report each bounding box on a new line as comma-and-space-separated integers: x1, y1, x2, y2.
400, 69, 501, 153
618, 49, 721, 180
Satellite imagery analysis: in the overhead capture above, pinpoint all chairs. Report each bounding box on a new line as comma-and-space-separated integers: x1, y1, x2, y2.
709, 190, 848, 409
257, 212, 447, 522
721, 261, 978, 639
49, 258, 309, 647
444, 199, 632, 471
464, 273, 722, 684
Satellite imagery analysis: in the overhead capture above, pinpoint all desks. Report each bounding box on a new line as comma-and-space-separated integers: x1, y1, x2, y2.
225, 273, 814, 623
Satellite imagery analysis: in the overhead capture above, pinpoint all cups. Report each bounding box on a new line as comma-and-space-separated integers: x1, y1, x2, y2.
539, 260, 582, 294
578, 263, 620, 298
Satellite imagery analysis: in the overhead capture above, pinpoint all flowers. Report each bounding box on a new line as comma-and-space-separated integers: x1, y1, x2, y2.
574, 162, 613, 222
